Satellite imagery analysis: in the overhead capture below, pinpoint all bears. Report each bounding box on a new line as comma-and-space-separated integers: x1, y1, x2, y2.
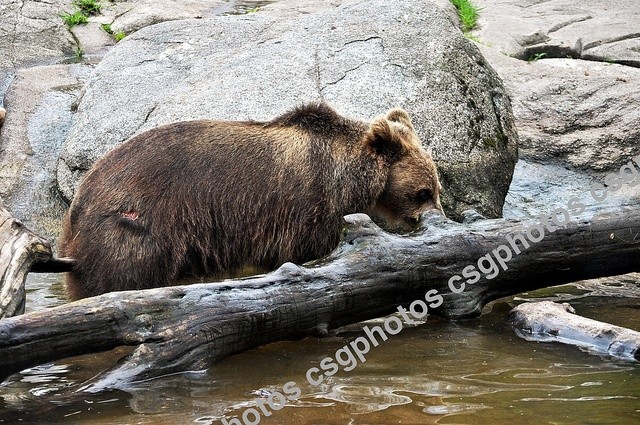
55, 101, 447, 302
